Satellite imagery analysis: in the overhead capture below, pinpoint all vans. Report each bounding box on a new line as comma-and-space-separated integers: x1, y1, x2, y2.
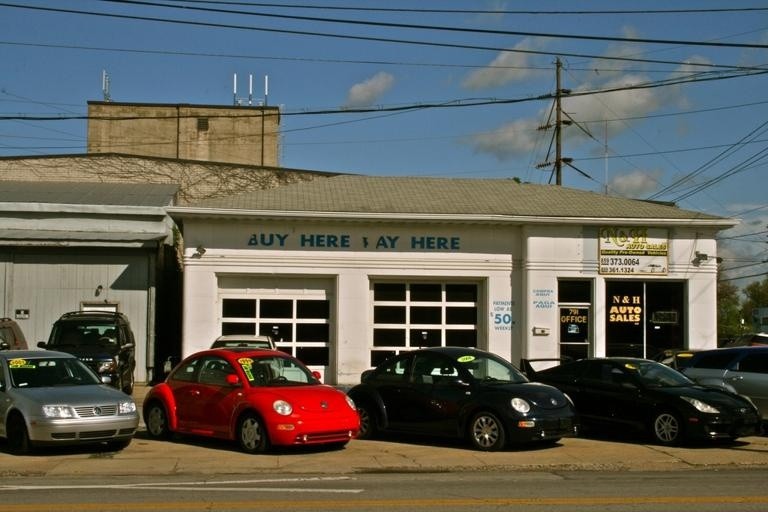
660, 345, 768, 435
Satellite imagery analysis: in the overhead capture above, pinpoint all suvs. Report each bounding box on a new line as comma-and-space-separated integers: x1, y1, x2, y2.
35, 309, 136, 393
0, 317, 28, 349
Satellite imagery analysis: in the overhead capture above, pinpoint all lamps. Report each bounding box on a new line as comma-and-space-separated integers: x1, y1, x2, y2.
696, 254, 707, 261
196, 245, 206, 254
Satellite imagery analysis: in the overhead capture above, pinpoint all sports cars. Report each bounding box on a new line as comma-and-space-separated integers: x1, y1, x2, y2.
518, 355, 760, 448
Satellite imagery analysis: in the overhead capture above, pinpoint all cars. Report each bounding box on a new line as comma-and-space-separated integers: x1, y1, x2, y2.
213, 334, 282, 378
143, 345, 361, 451
0, 348, 139, 451
348, 345, 576, 452
